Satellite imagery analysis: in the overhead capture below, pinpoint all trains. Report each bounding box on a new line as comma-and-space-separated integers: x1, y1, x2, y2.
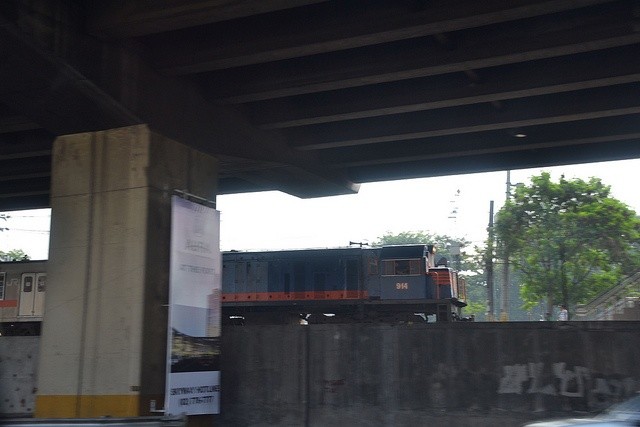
0, 243, 470, 335
